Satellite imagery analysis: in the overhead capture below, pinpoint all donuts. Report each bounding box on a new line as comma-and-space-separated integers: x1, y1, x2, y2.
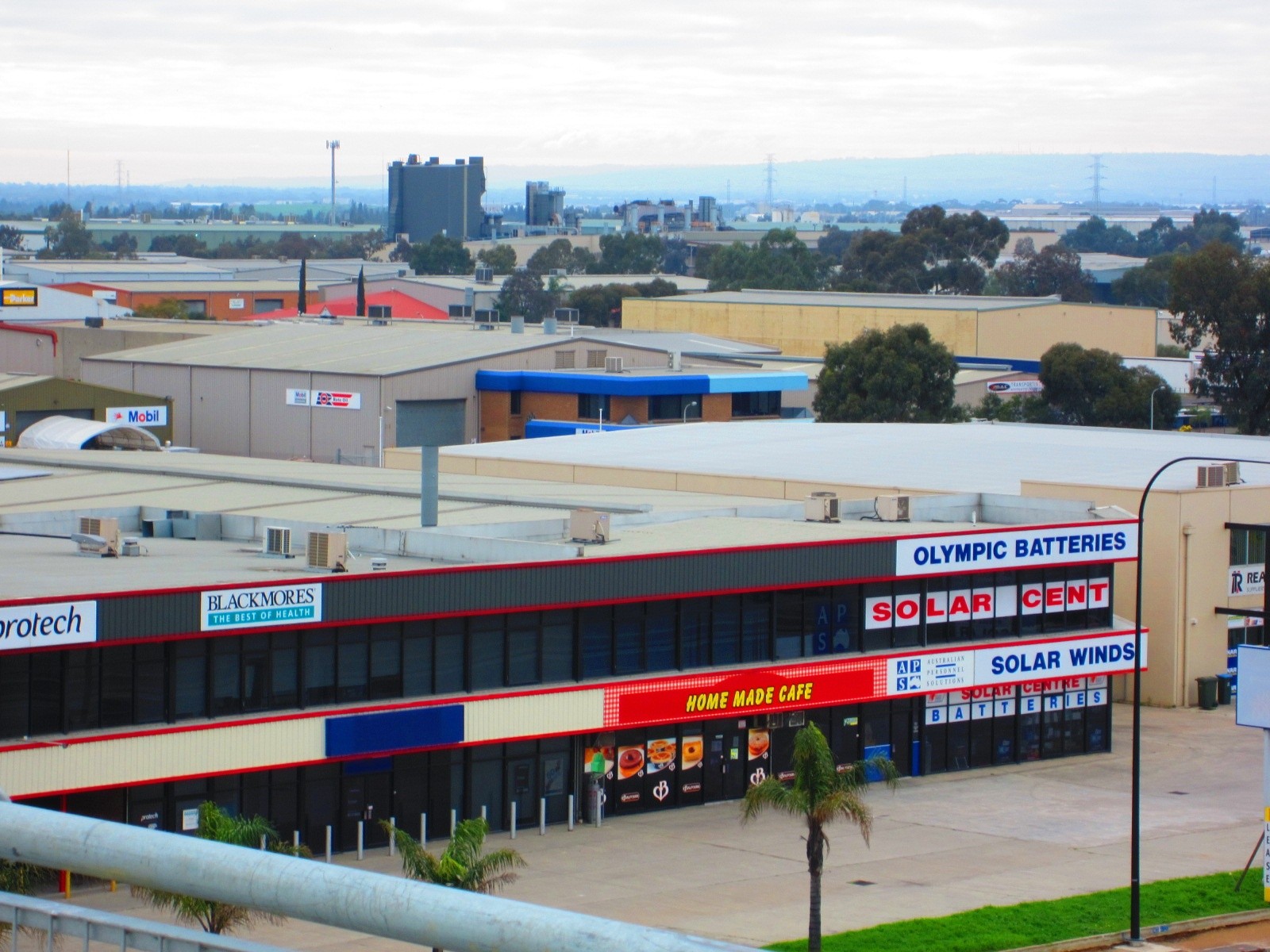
620, 750, 642, 768
749, 733, 768, 750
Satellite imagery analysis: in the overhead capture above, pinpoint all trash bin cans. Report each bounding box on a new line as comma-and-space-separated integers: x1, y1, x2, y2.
1195, 677, 1218, 708
1216, 674, 1233, 705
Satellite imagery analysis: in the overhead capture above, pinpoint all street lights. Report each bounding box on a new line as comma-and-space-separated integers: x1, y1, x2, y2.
326, 139, 339, 223
683, 400, 697, 425
1150, 385, 1166, 430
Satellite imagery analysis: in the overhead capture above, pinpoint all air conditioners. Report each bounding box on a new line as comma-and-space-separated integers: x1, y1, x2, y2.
570, 509, 610, 542
878, 495, 912, 521
77, 516, 119, 556
605, 357, 623, 373
1196, 460, 1241, 488
262, 525, 291, 555
305, 530, 348, 570
804, 495, 841, 522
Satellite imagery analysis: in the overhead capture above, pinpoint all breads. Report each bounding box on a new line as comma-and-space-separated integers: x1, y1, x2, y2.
646, 741, 676, 764
683, 740, 703, 762
